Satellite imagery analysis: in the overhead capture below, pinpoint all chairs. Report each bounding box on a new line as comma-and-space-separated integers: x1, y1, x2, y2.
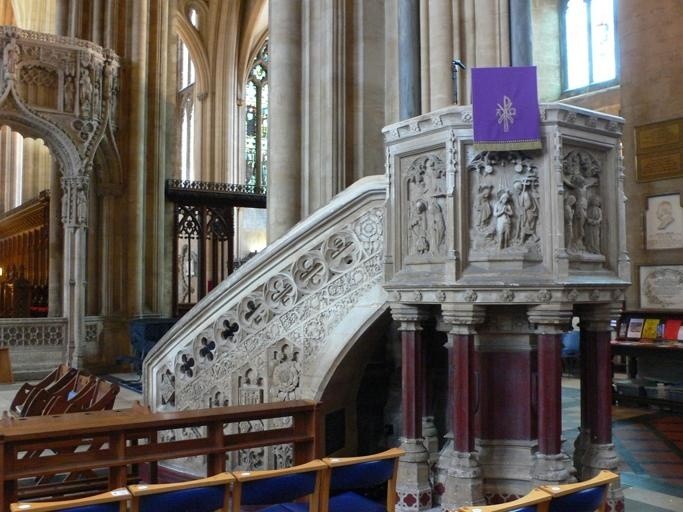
12, 485, 132, 512
126, 470, 236, 511
9, 364, 120, 417
232, 459, 327, 511
460, 488, 553, 512
539, 470, 620, 511
320, 448, 406, 511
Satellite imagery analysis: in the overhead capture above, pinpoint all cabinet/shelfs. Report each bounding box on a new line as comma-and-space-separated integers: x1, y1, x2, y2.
0, 399, 327, 511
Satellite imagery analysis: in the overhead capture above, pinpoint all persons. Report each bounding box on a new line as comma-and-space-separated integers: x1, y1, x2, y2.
403, 152, 603, 255
654, 200, 674, 232
65, 68, 100, 119
1, 36, 20, 82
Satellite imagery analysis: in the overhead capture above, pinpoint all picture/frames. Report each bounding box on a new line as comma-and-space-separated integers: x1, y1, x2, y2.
637, 263, 683, 311
640, 191, 683, 251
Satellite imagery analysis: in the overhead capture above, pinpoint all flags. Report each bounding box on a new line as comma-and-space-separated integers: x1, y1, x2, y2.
468, 66, 542, 153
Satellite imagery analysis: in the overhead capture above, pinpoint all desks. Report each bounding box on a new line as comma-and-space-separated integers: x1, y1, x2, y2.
611, 341, 683, 411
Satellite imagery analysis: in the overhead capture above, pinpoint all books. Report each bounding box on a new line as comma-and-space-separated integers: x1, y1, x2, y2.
624, 314, 682, 342
